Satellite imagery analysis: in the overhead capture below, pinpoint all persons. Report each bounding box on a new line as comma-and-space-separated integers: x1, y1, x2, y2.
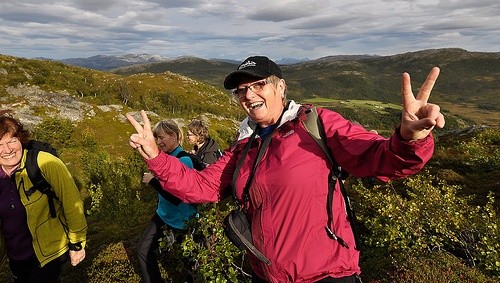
0, 117, 88, 283
134, 119, 202, 283
186, 122, 223, 170
125, 55, 445, 283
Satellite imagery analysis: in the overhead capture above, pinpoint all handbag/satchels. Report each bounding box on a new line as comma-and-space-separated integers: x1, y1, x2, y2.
222, 210, 271, 265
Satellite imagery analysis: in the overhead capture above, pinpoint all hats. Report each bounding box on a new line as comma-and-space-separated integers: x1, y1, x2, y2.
223, 55, 282, 90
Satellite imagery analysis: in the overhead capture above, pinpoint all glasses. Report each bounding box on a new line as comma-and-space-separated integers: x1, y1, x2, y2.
186, 131, 195, 136
232, 80, 269, 98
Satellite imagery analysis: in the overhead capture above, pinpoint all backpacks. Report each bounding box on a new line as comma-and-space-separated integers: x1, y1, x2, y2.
176, 150, 203, 171
25, 139, 59, 218
